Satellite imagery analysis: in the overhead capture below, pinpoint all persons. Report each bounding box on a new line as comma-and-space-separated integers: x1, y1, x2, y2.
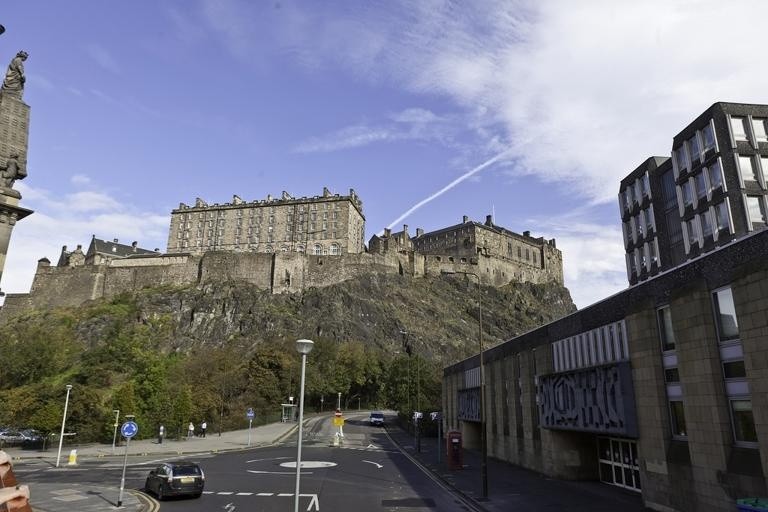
187, 421, 195, 438
159, 422, 164, 443
0, 153, 20, 186
200, 419, 207, 437
3, 50, 29, 90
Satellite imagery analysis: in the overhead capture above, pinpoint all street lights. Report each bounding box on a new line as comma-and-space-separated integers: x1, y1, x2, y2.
338, 392, 342, 408
394, 330, 420, 420
247, 408, 254, 445
293, 339, 314, 512
440, 271, 492, 500
111, 410, 136, 505
55, 384, 74, 466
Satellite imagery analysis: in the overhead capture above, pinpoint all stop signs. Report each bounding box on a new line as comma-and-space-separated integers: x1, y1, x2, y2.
335, 410, 341, 417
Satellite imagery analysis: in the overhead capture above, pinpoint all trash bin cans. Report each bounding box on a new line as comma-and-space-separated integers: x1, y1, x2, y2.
333, 436, 339, 447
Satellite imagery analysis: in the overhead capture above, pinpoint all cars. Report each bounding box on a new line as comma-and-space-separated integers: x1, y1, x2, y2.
0, 427, 44, 447
145, 462, 205, 500
370, 411, 385, 426
